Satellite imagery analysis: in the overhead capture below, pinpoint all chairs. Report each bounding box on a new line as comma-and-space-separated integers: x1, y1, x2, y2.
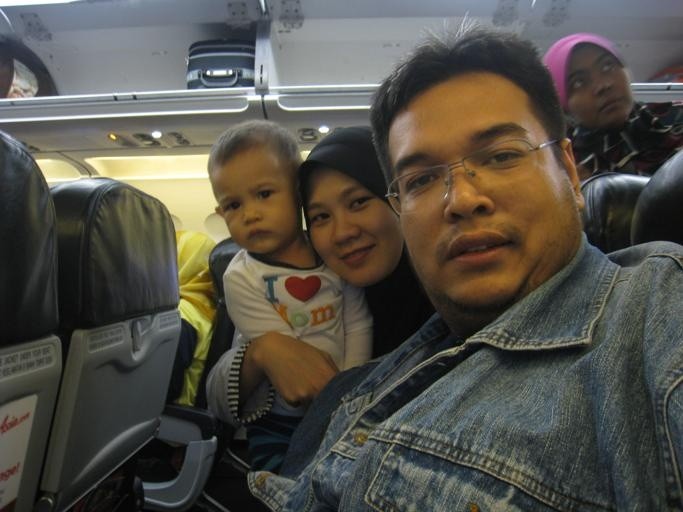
0, 132, 64, 512
33, 177, 217, 512
195, 237, 242, 409
576, 172, 650, 254
630, 149, 683, 245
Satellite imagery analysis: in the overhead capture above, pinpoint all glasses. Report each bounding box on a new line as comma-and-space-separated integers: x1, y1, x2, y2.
384, 139, 560, 215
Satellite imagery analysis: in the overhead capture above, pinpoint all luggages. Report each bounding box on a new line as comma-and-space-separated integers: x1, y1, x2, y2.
187, 39, 255, 90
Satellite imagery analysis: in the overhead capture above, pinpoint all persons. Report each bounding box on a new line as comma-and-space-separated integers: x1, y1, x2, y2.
185, 128, 440, 512
207, 121, 376, 471
540, 30, 683, 182
247, 19, 682, 512
